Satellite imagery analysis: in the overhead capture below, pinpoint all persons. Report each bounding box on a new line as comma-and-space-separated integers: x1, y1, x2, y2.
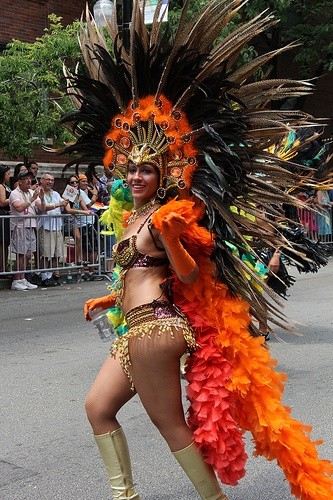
293, 160, 332, 252
125, 196, 159, 226
0, 160, 116, 292
243, 178, 289, 343
80, 143, 228, 500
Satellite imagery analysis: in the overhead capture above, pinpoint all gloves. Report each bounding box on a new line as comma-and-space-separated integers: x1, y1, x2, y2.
161, 212, 195, 276
83, 292, 117, 322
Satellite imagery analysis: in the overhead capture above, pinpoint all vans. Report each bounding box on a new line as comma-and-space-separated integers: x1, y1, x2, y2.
0, 160, 104, 199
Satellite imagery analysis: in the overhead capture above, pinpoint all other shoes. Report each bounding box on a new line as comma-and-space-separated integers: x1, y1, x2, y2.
19, 278, 37, 290
77, 260, 86, 266
259, 332, 270, 341
10, 280, 27, 290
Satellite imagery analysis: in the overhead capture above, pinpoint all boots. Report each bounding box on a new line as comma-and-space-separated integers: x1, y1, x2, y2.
171, 440, 228, 500
92, 427, 139, 499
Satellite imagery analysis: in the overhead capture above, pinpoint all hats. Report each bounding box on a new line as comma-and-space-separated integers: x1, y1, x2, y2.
16, 171, 33, 178
78, 174, 86, 180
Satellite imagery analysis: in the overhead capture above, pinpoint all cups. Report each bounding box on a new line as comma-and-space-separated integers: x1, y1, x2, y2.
89, 308, 118, 344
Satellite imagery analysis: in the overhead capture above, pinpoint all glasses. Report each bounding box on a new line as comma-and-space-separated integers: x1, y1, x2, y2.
69, 180, 78, 184
